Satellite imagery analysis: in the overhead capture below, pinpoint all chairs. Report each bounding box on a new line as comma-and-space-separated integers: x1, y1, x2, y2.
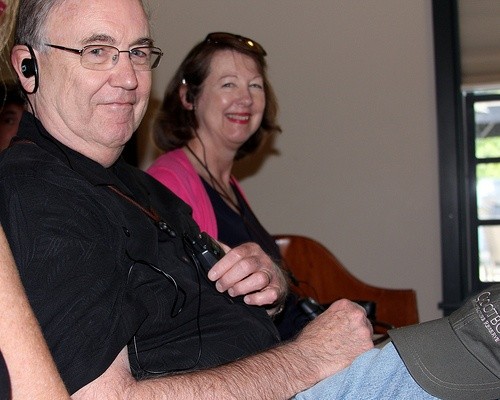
272, 234, 420, 336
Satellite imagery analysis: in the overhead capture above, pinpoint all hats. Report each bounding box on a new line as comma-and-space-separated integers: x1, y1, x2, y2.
389, 284, 500, 400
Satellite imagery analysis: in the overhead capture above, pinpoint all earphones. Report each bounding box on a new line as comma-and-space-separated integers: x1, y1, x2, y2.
21, 58, 34, 78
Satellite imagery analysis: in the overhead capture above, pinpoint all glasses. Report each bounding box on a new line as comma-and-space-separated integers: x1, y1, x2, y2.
44, 43, 163, 71
185, 32, 266, 72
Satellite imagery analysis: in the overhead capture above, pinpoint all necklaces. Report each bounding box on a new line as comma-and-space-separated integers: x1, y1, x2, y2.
185, 143, 250, 217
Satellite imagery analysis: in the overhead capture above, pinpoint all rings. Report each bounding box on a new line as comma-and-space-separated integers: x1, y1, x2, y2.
260, 270, 273, 284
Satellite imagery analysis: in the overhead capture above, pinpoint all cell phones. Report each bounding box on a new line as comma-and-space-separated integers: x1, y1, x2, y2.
184, 232, 225, 273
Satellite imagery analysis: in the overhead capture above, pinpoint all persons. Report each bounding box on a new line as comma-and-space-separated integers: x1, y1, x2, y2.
144, 31, 281, 265
0, 0, 441, 400
